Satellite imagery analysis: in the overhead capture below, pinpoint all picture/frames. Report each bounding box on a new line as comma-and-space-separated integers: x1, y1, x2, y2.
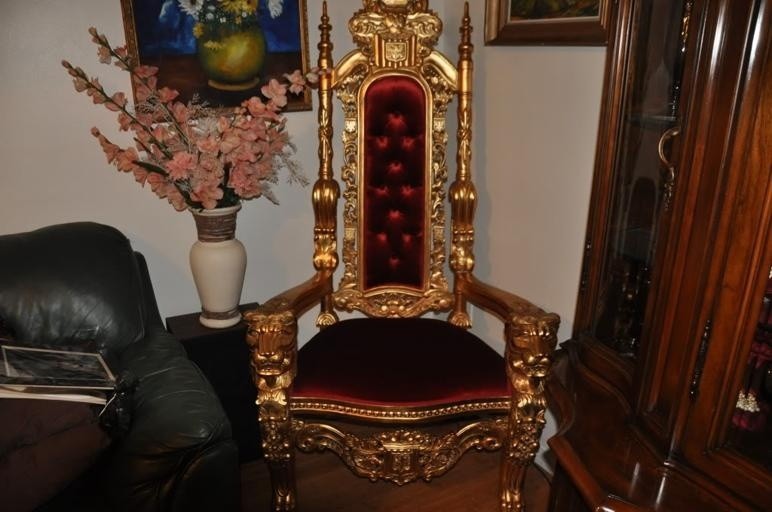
483, 0, 613, 45
119, 0, 312, 123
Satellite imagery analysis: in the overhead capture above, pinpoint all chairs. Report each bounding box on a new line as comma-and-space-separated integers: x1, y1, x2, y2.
0, 222, 242, 512
242, 0, 561, 512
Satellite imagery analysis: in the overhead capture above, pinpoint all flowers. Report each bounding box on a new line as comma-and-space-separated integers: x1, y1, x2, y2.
61, 26, 320, 212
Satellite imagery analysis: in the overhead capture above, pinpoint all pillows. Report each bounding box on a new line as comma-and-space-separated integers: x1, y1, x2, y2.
0, 223, 148, 365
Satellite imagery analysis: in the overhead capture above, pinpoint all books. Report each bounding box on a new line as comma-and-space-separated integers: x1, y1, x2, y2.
0, 337, 117, 406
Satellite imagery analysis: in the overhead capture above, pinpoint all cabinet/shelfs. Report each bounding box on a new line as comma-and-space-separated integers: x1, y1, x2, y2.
166, 302, 260, 464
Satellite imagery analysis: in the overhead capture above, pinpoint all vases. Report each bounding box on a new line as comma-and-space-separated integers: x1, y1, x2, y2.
188, 202, 248, 329
